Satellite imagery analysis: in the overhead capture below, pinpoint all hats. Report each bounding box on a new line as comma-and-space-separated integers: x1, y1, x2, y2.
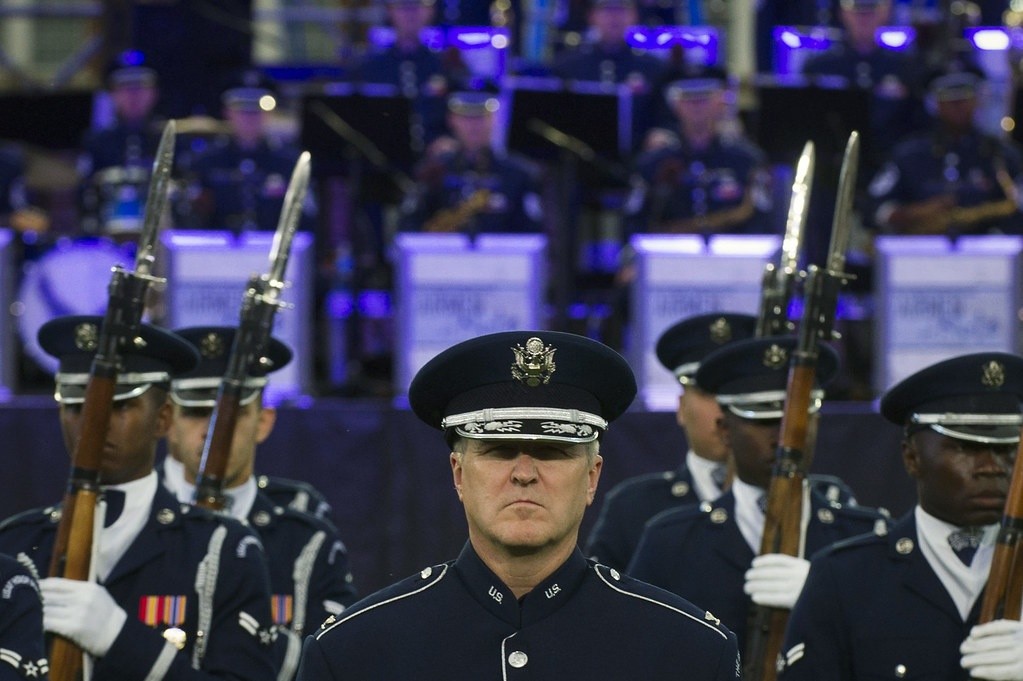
879, 352, 1023, 443
161, 326, 294, 406
923, 58, 988, 102
446, 76, 500, 116
654, 313, 780, 385
695, 336, 838, 419
655, 64, 730, 105
100, 46, 159, 92
219, 67, 278, 113
36, 315, 201, 404
409, 329, 638, 443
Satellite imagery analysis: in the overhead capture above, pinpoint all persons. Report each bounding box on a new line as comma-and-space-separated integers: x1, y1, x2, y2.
164, 327, 356, 661
289, 331, 743, 681
583, 311, 759, 573
621, 337, 895, 680
0, 316, 278, 681
0, 0, 1023, 241
776, 350, 1023, 681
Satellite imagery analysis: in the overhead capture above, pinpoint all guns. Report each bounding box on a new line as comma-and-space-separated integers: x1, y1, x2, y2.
194, 151, 312, 508
977, 430, 1023, 624
741, 130, 861, 681
46, 119, 179, 681
759, 140, 816, 341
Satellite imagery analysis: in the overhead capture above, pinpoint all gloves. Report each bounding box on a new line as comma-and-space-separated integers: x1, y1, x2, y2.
959, 619, 1023, 681
30, 577, 128, 658
743, 553, 812, 611
